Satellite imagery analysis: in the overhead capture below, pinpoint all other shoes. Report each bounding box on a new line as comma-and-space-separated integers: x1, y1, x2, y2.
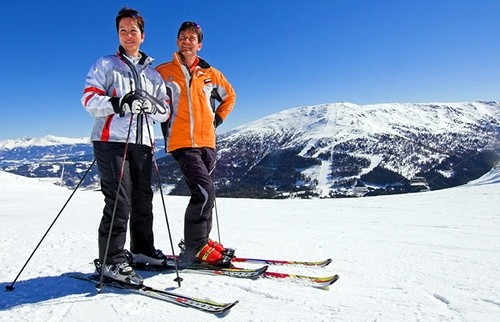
131, 249, 166, 264
102, 258, 144, 286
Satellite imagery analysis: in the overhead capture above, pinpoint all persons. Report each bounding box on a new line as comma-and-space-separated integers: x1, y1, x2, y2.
80, 7, 174, 288
154, 20, 236, 267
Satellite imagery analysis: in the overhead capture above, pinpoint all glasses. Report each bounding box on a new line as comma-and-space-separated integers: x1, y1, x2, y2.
182, 21, 199, 30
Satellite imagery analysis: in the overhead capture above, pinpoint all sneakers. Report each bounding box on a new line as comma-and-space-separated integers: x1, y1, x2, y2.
187, 243, 223, 264
207, 239, 224, 253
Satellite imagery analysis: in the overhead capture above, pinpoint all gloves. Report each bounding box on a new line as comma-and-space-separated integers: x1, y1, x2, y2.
215, 115, 222, 128
140, 97, 153, 114
119, 91, 145, 114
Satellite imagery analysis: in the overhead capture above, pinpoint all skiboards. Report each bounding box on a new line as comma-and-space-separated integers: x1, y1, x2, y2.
61, 265, 269, 313
166, 254, 339, 288
